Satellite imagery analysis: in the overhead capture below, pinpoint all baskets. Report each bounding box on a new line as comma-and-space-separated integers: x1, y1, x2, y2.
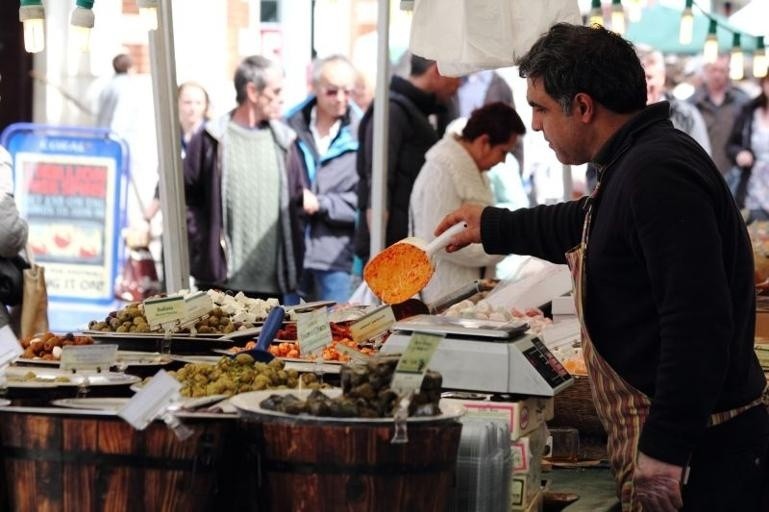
553, 375, 607, 461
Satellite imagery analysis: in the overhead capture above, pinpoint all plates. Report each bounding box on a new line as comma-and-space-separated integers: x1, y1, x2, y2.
231, 388, 467, 424
8, 347, 176, 366
0, 368, 140, 387
51, 396, 243, 416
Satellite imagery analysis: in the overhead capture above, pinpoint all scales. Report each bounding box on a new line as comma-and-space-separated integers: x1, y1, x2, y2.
379, 314, 576, 397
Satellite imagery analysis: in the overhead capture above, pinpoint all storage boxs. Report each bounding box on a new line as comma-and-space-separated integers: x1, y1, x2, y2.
437, 387, 556, 512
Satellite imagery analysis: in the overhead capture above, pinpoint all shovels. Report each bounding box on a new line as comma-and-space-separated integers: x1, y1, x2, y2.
230, 306, 285, 364
362, 220, 470, 305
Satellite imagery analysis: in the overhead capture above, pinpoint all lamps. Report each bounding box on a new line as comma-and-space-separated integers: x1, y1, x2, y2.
588, 0, 769, 79
17, 0, 54, 58
66, 0, 100, 32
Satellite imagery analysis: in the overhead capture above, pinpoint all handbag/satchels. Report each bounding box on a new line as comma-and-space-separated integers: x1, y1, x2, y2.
18, 240, 48, 347
114, 218, 160, 301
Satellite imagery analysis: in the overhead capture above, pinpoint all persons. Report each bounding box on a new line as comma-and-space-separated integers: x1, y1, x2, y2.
91, 51, 530, 315
0, 144, 31, 375
432, 18, 769, 510
636, 45, 769, 226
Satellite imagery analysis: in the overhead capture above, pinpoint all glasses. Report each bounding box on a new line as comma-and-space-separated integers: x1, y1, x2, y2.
325, 87, 352, 97
